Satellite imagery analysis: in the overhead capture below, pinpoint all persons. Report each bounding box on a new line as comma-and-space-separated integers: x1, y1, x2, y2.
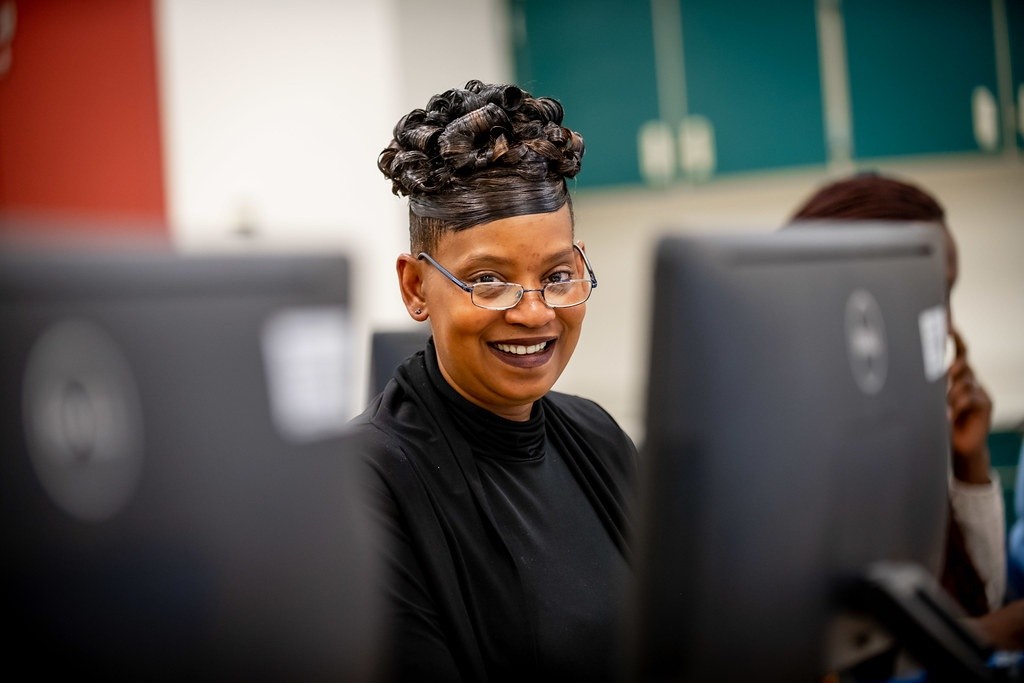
346, 79, 644, 683
781, 173, 1024, 683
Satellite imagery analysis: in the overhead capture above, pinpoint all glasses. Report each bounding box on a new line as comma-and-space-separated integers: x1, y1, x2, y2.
418, 244, 597, 311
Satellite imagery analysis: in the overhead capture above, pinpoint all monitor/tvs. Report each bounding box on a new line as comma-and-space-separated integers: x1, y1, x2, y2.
0, 233, 389, 683
638, 223, 991, 683
